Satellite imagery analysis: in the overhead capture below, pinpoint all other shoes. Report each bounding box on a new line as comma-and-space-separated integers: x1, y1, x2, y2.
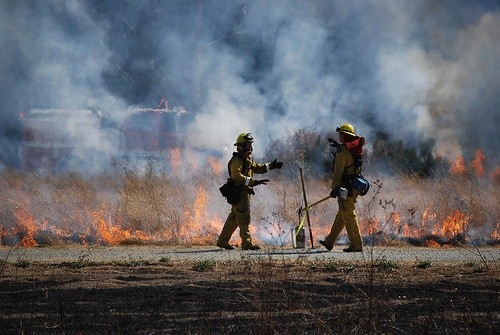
343, 247, 363, 252
319, 240, 333, 251
243, 244, 261, 250
217, 244, 234, 250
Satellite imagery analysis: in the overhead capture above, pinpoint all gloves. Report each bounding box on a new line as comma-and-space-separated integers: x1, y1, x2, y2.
254, 179, 270, 187
330, 188, 340, 198
328, 138, 339, 148
269, 159, 284, 171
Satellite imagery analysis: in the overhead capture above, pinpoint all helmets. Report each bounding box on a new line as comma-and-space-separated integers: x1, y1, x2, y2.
338, 124, 356, 136
234, 132, 254, 146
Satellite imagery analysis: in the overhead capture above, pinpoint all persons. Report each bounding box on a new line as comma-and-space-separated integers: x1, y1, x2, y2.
320, 124, 363, 252
216, 132, 284, 251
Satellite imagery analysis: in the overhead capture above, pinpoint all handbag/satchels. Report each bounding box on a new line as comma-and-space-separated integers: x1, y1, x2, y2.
348, 176, 370, 197
218, 182, 241, 205
